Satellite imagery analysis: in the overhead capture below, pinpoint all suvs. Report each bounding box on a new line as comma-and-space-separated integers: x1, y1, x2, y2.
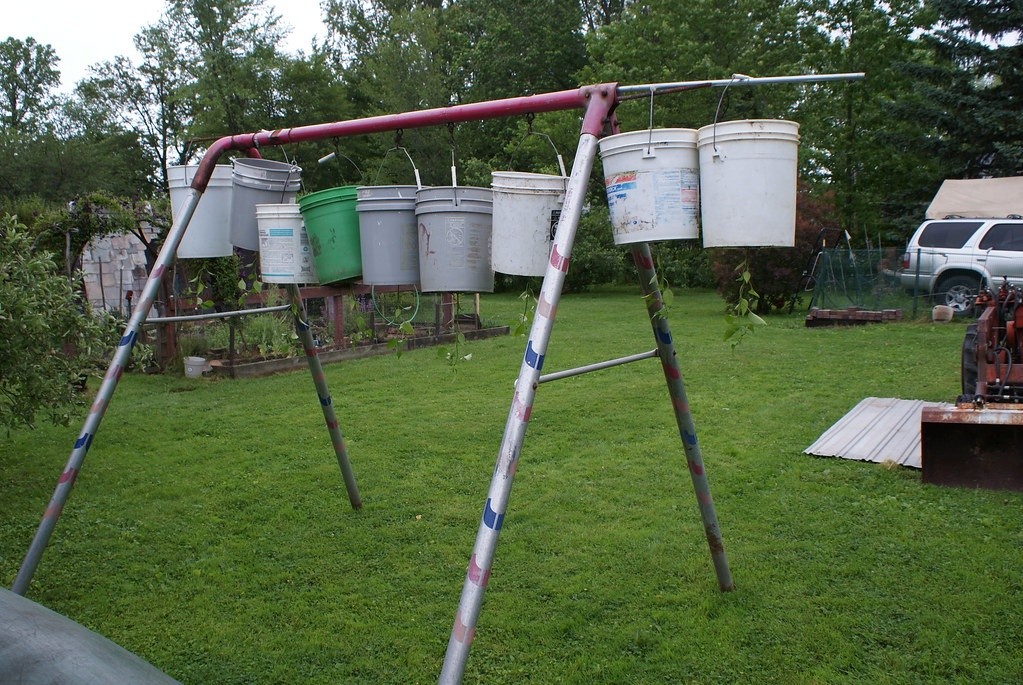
901, 214, 1023, 318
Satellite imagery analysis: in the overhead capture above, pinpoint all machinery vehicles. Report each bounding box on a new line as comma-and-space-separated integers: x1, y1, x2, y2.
919, 277, 1023, 491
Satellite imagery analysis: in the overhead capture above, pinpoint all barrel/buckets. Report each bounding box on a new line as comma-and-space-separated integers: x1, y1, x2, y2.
166, 137, 234, 259
597, 86, 698, 245
297, 152, 364, 287
183, 356, 206, 378
355, 146, 429, 286
415, 146, 496, 294
490, 131, 571, 276
229, 129, 302, 252
253, 168, 319, 284
697, 73, 802, 249
